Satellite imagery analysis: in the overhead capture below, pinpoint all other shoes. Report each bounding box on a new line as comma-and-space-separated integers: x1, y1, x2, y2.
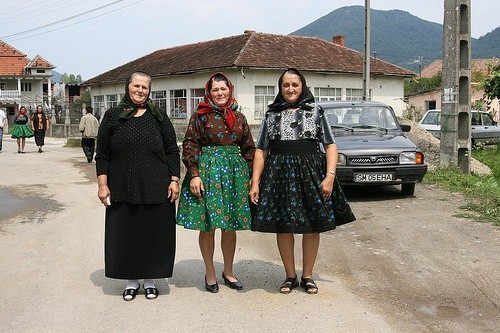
39, 149, 43, 153
87, 157, 93, 163
18, 151, 25, 153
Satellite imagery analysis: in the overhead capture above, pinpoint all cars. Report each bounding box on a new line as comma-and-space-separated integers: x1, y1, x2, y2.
315, 100, 428, 197
418, 109, 500, 147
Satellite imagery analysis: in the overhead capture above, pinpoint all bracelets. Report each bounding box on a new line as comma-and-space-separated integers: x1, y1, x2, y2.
171, 179, 180, 185
326, 170, 336, 177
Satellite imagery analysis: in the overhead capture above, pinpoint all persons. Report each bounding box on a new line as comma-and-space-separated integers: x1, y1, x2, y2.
95, 71, 180, 301
79, 107, 100, 162
11, 106, 34, 153
0, 103, 6, 151
31, 105, 48, 153
249, 68, 356, 293
176, 72, 256, 294
94, 112, 100, 121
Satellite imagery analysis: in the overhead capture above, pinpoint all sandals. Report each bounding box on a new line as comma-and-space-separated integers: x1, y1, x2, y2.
279, 276, 318, 295
122, 284, 159, 302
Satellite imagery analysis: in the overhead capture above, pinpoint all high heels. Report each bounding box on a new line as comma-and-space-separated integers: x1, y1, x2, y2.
205, 272, 243, 294
